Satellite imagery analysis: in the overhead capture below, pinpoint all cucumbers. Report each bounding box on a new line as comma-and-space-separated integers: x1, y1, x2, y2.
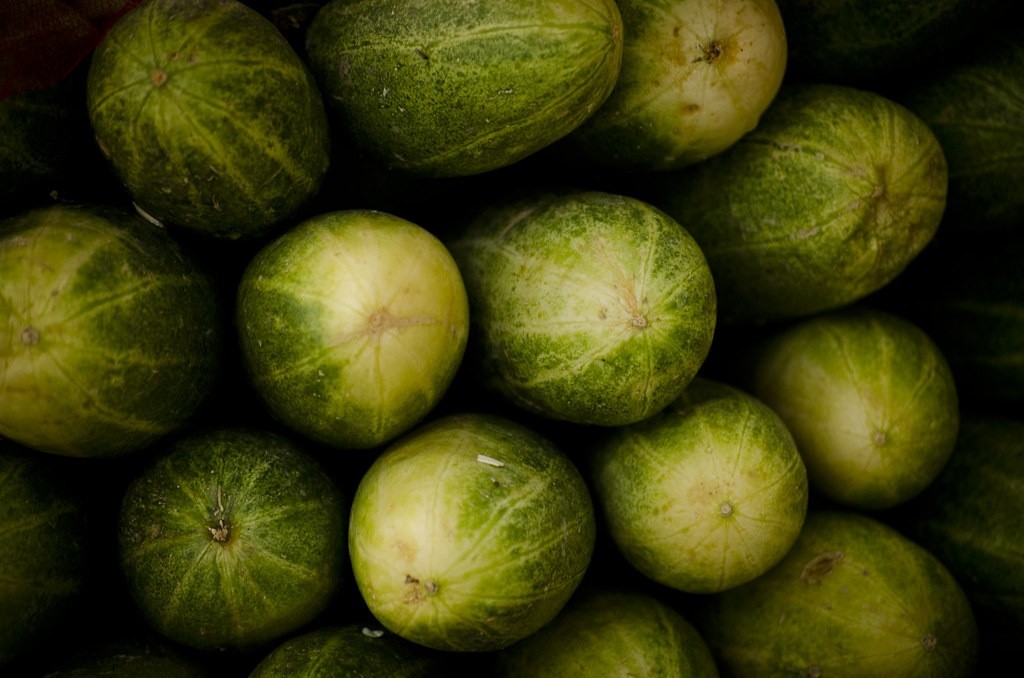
0, 0, 1024, 678
